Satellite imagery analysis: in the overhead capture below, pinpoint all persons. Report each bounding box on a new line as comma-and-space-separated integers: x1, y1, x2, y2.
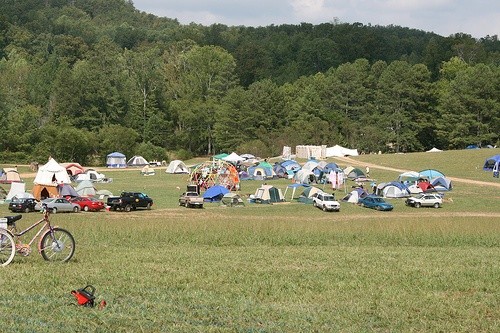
366, 166, 369, 177
249, 194, 264, 202
372, 183, 377, 194
58, 180, 64, 193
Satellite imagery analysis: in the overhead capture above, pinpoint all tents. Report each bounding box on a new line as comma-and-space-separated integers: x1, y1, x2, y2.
201, 185, 244, 208
283, 184, 309, 201
239, 153, 276, 180
465, 145, 480, 150
426, 148, 443, 152
188, 152, 240, 191
255, 184, 284, 203
165, 160, 189, 174
299, 186, 324, 204
482, 154, 500, 178
326, 144, 358, 157
271, 159, 453, 205
0, 156, 113, 201
106, 152, 126, 169
127, 156, 155, 177
486, 145, 493, 149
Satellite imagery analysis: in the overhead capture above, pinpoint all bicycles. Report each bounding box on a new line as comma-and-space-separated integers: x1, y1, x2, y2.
1, 204, 76, 267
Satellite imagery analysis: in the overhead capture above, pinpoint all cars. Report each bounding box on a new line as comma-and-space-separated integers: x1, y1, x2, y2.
67, 196, 105, 212
405, 193, 443, 209
9, 198, 40, 212
358, 196, 393, 212
34, 197, 80, 213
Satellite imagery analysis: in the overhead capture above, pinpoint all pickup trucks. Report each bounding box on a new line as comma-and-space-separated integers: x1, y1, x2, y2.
312, 192, 340, 212
107, 192, 154, 212
178, 191, 204, 207
